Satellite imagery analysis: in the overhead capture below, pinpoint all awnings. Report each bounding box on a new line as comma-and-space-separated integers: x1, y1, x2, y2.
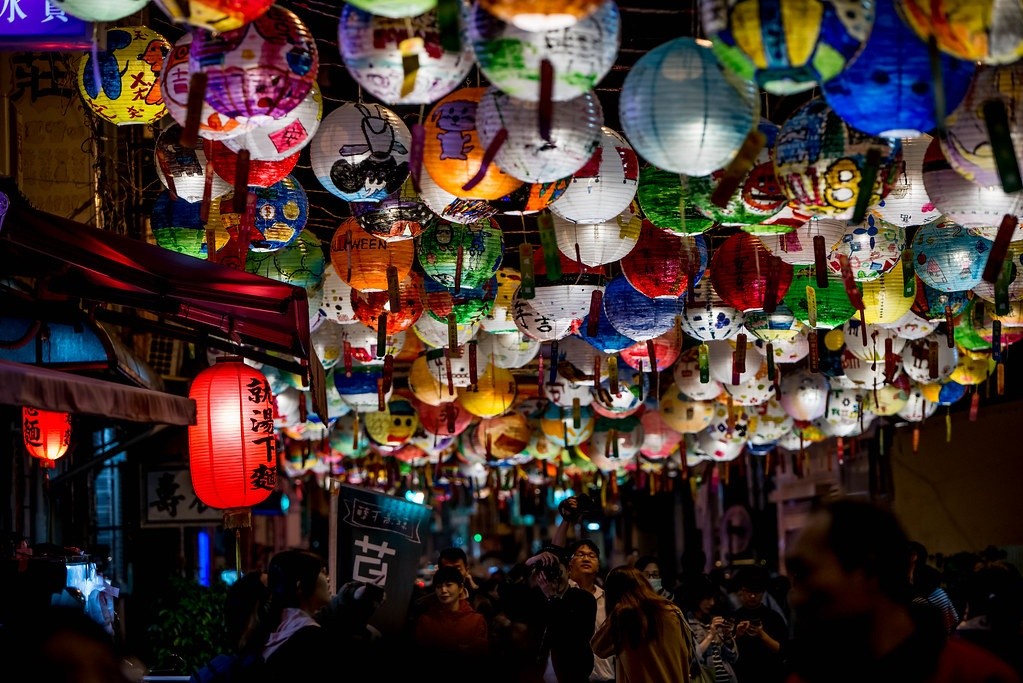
0, 360, 198, 485
0, 203, 329, 425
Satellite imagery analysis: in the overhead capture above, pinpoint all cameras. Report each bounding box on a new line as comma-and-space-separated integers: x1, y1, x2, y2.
535, 558, 561, 584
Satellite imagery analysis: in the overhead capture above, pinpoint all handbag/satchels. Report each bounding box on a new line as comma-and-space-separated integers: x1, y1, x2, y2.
671, 603, 715, 683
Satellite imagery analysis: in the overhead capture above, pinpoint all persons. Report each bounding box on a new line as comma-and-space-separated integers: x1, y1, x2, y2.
0, 485, 1023, 683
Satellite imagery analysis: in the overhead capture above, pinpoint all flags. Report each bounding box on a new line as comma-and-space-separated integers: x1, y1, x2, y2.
338, 482, 432, 610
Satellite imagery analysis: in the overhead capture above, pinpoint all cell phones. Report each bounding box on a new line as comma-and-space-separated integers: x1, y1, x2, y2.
750, 619, 760, 628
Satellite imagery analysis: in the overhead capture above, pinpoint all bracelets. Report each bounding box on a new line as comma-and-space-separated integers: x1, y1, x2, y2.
547, 595, 560, 602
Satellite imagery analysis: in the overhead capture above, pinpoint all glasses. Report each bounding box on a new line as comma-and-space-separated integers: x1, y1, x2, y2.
643, 573, 659, 580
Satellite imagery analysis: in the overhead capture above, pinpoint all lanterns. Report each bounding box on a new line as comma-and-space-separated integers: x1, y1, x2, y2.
49, 0, 1023, 489
22, 406, 72, 468
188, 355, 276, 529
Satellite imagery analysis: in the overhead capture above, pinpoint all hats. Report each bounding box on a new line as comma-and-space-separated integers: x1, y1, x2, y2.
534, 547, 569, 568
